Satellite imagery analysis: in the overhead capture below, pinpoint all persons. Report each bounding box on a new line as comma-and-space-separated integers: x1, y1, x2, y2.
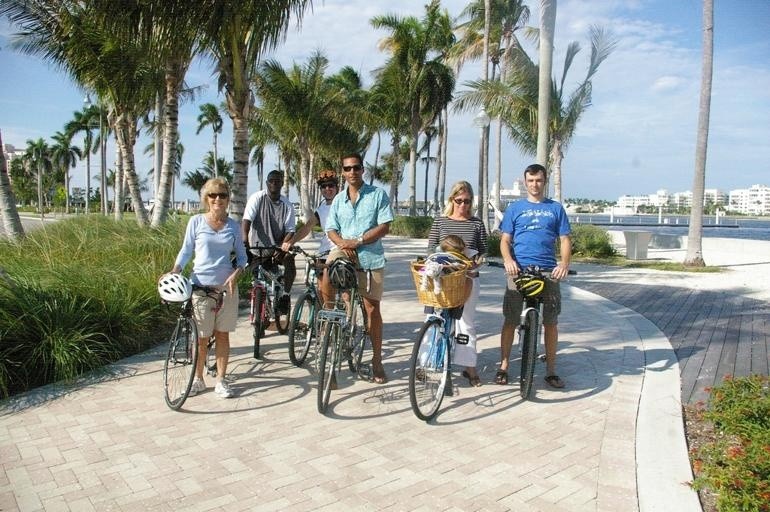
157, 177, 248, 399
241, 168, 296, 330
282, 170, 356, 336
496, 163, 574, 388
436, 235, 480, 275
322, 153, 388, 385
418, 180, 482, 387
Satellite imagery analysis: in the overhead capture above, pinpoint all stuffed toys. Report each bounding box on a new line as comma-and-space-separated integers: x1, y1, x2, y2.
421, 259, 443, 295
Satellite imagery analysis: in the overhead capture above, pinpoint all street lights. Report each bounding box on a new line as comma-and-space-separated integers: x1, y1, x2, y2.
83, 89, 105, 217
474, 106, 491, 223
32, 146, 42, 214
276, 145, 284, 170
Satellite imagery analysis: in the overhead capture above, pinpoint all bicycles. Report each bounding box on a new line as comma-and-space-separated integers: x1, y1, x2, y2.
409, 243, 499, 420
159, 283, 229, 411
482, 255, 579, 404
303, 255, 368, 414
229, 241, 290, 362
287, 242, 331, 370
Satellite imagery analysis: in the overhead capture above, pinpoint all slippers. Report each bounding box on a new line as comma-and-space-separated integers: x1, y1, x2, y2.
463, 370, 482, 388
373, 368, 384, 384
544, 375, 565, 388
496, 370, 508, 384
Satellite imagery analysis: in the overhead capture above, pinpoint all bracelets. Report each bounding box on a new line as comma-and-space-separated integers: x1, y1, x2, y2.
358, 236, 364, 244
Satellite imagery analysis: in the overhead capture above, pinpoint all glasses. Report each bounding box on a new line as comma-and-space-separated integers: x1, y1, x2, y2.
321, 183, 336, 188
343, 165, 361, 172
453, 199, 471, 204
208, 193, 229, 199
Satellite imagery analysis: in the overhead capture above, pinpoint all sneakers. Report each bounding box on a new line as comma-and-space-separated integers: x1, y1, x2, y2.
215, 382, 234, 398
181, 377, 207, 397
279, 293, 290, 315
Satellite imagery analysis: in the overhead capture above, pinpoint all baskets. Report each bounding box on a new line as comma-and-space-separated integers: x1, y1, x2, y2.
410, 260, 466, 309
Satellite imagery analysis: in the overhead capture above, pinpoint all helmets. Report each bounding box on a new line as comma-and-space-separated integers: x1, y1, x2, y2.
513, 271, 546, 300
329, 258, 358, 290
316, 171, 339, 184
158, 274, 192, 303
